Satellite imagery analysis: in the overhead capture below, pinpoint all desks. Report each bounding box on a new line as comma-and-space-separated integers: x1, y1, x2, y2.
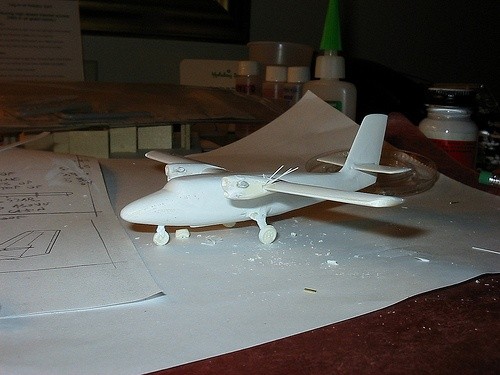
1, 145, 499, 375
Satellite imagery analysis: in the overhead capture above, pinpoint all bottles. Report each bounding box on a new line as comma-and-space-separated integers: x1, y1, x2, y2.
261, 65, 287, 98
283, 66, 310, 106
236, 61, 261, 95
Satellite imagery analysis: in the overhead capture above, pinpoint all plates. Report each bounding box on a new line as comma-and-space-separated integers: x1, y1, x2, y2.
305, 148, 437, 197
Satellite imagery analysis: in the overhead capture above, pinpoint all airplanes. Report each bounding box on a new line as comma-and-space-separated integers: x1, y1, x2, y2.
120, 113, 411, 245
